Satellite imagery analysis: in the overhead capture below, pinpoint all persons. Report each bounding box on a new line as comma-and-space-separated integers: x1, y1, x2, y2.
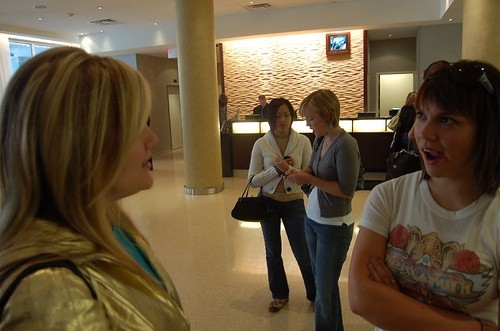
253, 95, 269, 118
247, 97, 316, 313
386, 91, 418, 180
0, 48, 190, 331
219, 93, 237, 134
349, 59, 500, 331
286, 89, 359, 331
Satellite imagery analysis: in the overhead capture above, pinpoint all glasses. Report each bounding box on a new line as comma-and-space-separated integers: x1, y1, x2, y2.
424, 60, 494, 97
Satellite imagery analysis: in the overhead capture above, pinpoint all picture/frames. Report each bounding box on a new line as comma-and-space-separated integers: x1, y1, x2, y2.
326, 32, 350, 55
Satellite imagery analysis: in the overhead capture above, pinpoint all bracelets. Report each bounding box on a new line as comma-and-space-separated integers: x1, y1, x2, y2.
476, 319, 483, 331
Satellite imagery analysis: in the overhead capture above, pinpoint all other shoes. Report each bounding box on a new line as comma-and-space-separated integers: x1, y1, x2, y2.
269, 298, 289, 312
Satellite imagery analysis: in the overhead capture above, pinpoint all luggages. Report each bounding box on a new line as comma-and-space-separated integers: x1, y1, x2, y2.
384, 136, 419, 180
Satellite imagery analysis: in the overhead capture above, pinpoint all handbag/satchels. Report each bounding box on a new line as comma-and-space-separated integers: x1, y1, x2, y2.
335, 144, 365, 190
230, 176, 267, 220
